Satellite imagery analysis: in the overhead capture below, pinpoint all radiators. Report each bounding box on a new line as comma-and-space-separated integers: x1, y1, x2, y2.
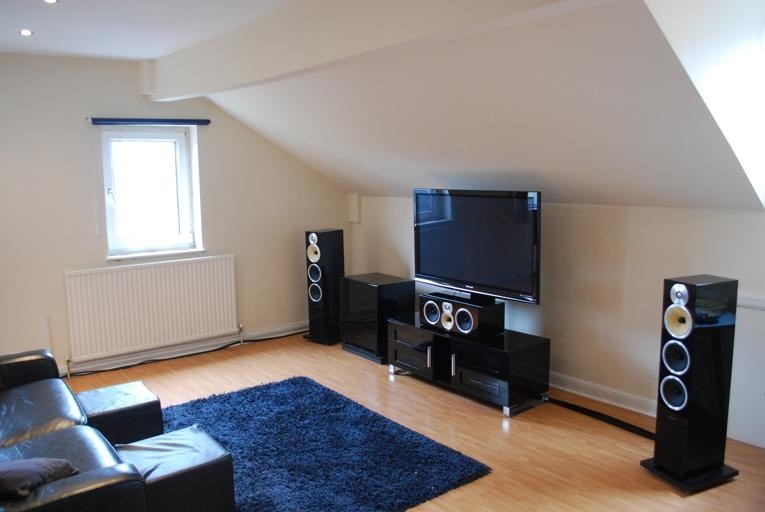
62, 252, 245, 380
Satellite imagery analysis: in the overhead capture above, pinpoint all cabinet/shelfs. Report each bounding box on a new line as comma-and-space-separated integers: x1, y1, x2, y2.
386, 308, 552, 418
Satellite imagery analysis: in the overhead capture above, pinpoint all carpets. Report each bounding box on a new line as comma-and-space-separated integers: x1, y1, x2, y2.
160, 374, 494, 511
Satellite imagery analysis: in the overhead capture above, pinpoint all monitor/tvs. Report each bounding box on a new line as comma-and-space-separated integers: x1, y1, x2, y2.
411, 188, 541, 310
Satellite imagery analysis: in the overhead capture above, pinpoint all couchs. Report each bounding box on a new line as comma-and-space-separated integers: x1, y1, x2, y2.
0, 346, 152, 510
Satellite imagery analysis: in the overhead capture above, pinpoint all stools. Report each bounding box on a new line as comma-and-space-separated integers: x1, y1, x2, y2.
74, 379, 165, 444
117, 423, 238, 512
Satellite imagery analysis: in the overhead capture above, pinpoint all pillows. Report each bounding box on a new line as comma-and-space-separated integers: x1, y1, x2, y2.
0, 456, 81, 497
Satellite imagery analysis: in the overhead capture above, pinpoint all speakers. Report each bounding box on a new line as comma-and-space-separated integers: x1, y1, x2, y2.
655, 272, 737, 480
418, 290, 505, 344
303, 228, 345, 345
